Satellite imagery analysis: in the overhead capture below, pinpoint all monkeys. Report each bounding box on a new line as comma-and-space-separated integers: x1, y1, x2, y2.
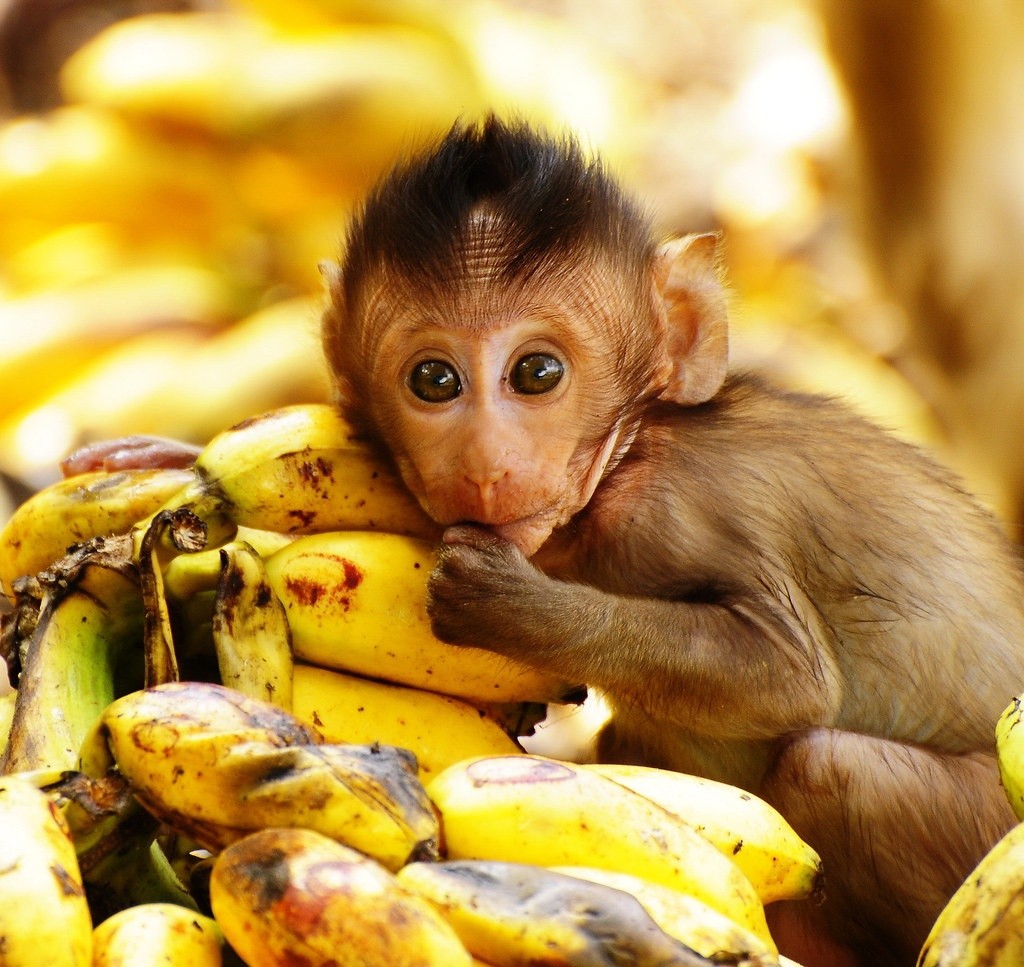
61, 108, 1024, 967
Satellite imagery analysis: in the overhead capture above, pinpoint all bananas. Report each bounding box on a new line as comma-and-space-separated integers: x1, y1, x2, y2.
0, 0, 1024, 967
